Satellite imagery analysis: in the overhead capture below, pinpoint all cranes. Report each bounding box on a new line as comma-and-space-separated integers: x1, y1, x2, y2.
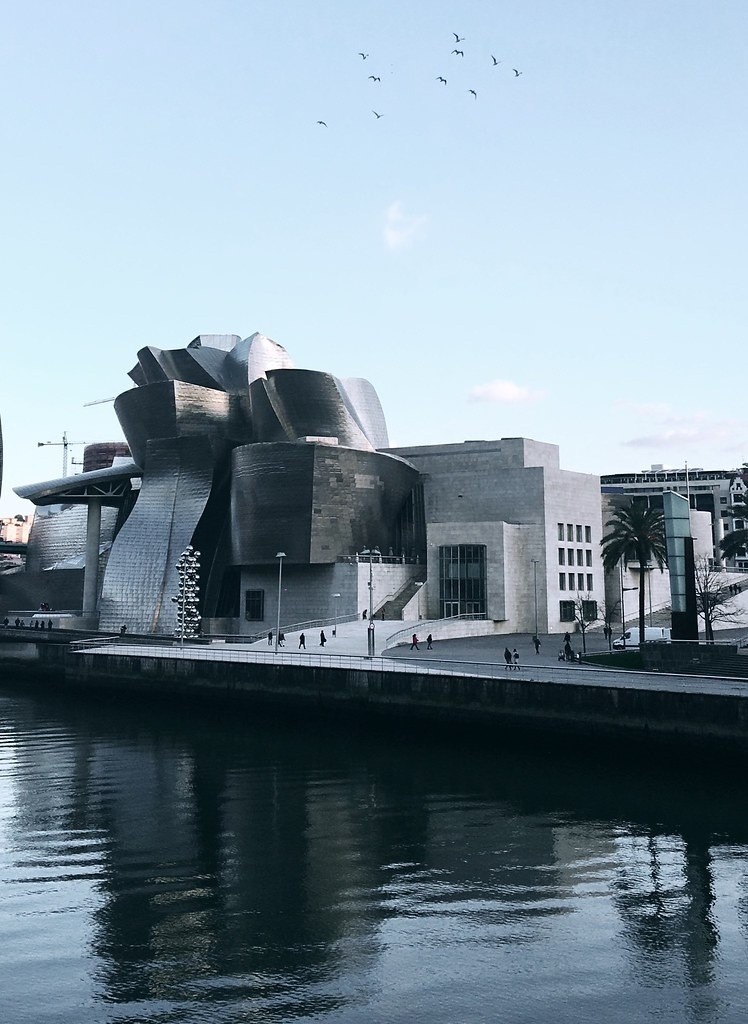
37, 431, 89, 477
83, 396, 117, 407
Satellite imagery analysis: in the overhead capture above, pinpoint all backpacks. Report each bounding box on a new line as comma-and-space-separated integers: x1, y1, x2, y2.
514, 653, 519, 658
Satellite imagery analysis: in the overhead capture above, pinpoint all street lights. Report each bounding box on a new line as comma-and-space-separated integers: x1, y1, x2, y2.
646, 564, 653, 627
334, 593, 340, 638
275, 552, 287, 655
530, 558, 540, 639
359, 549, 381, 658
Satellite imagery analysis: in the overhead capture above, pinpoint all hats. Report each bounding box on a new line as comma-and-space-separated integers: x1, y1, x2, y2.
513, 649, 517, 652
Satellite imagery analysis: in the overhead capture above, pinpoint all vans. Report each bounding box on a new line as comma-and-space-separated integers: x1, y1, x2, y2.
612, 626, 665, 650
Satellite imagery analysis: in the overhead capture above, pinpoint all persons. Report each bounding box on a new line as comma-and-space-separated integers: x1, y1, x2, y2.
41, 602, 49, 611
729, 584, 741, 595
410, 634, 420, 650
320, 630, 325, 647
534, 639, 541, 653
427, 634, 433, 649
363, 610, 367, 619
604, 625, 612, 640
565, 643, 571, 659
563, 632, 570, 642
121, 625, 127, 634
268, 632, 273, 645
30, 619, 53, 631
512, 649, 520, 670
299, 633, 306, 649
15, 618, 25, 630
504, 648, 511, 670
4, 618, 9, 628
278, 631, 285, 647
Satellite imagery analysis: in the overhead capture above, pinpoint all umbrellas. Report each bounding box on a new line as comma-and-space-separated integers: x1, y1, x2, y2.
360, 549, 381, 554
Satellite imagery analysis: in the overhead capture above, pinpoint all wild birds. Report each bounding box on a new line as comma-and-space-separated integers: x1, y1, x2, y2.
491, 56, 500, 66
451, 49, 464, 57
368, 75, 380, 82
435, 77, 447, 85
358, 53, 369, 60
469, 90, 476, 96
317, 120, 328, 127
372, 110, 384, 119
453, 33, 465, 42
512, 69, 523, 77
